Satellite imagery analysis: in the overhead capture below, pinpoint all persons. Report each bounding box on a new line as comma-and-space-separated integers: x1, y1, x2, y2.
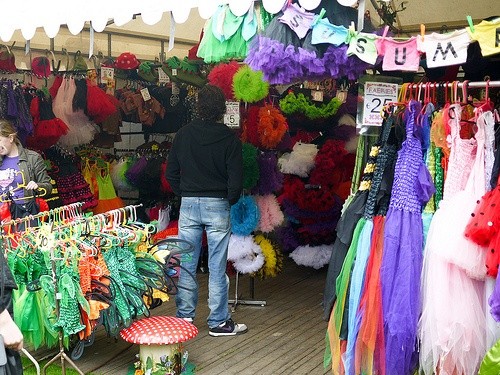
0, 119, 52, 217
0, 250, 25, 375
166, 85, 249, 336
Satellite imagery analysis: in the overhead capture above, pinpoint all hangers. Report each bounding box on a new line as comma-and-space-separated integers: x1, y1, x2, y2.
1, 202, 156, 277
380, 75, 494, 127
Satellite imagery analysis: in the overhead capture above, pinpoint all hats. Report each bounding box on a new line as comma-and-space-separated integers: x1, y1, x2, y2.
114, 52, 141, 70
31, 56, 50, 80
0, 51, 17, 72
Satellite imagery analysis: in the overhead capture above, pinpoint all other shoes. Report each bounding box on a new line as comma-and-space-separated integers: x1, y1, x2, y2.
137, 56, 206, 88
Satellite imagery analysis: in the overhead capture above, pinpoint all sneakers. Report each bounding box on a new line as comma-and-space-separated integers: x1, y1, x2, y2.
182, 317, 194, 326
209, 320, 249, 337
